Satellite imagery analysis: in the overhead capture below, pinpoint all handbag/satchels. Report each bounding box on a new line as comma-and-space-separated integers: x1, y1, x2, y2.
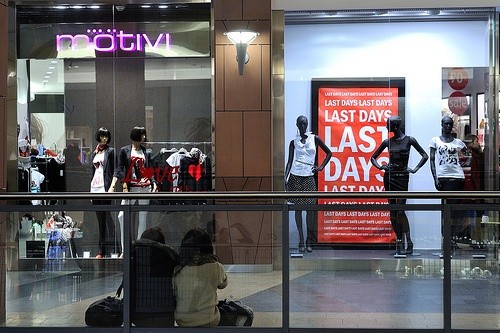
84, 295, 123, 327
218, 295, 255, 327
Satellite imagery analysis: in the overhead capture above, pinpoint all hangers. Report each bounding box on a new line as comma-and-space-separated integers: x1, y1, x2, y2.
190, 142, 199, 154
175, 145, 188, 154
167, 144, 178, 151
159, 142, 166, 154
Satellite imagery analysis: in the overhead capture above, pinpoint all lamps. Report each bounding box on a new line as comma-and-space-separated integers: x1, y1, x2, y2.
224, 29, 261, 74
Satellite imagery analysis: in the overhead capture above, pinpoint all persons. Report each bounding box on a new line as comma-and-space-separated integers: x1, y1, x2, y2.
89, 127, 118, 259
371, 116, 429, 255
123, 228, 178, 328
284, 115, 332, 252
462, 135, 482, 249
429, 115, 467, 256
115, 125, 158, 259
171, 227, 228, 328
